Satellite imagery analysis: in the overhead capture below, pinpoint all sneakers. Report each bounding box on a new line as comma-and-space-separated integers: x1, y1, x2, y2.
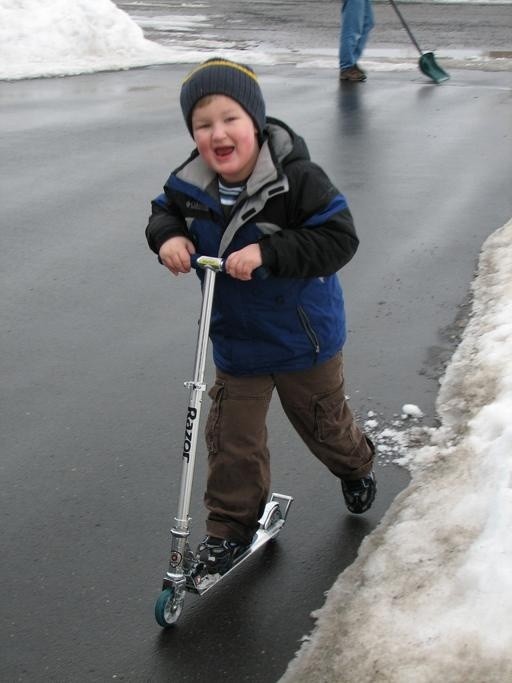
338, 467, 379, 513
339, 64, 368, 82
195, 532, 261, 573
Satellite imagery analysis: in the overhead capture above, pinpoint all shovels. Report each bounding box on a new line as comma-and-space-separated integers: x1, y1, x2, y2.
389, 0, 450, 83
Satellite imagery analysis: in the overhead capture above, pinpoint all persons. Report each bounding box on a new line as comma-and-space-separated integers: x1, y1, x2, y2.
143, 55, 378, 579
337, 0, 376, 80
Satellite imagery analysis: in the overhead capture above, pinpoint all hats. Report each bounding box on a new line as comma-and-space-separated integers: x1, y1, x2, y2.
180, 55, 265, 139
